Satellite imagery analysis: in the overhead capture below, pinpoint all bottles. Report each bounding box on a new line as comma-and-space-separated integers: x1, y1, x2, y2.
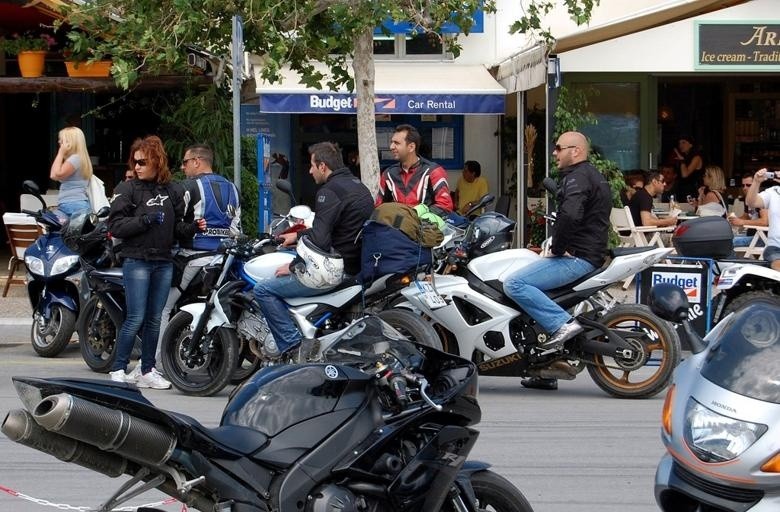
735, 120, 759, 137
669, 195, 675, 212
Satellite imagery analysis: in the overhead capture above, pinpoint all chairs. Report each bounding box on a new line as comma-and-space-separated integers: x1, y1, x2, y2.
608, 205, 673, 290
5, 224, 46, 298
732, 224, 770, 261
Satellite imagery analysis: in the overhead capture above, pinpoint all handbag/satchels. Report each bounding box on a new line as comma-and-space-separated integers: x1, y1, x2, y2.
369, 202, 443, 246
355, 220, 434, 280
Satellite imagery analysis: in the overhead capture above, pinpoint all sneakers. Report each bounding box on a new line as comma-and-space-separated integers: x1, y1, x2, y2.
130, 364, 173, 390
542, 319, 585, 349
111, 369, 130, 384
520, 375, 560, 391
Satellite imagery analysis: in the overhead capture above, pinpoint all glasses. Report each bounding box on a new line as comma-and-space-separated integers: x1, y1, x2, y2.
741, 183, 753, 188
133, 159, 147, 166
656, 179, 665, 184
555, 144, 576, 151
183, 156, 202, 166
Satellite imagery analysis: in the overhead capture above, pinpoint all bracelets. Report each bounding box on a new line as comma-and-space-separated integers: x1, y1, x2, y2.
468, 201, 474, 209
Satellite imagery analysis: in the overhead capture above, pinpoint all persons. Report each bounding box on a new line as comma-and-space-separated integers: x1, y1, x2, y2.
502, 132, 613, 390
125, 142, 243, 382
106, 134, 206, 390
619, 135, 780, 272
50, 126, 94, 218
270, 151, 290, 180
452, 159, 488, 222
252, 139, 375, 365
374, 124, 453, 222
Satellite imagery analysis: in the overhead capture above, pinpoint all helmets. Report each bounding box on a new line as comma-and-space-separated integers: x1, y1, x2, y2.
467, 212, 516, 253
292, 235, 347, 291
62, 210, 110, 253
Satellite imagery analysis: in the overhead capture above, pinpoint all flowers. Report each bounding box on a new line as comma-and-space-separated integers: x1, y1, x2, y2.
1, 30, 55, 55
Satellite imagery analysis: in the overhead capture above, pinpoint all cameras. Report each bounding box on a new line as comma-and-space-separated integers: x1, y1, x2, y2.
686, 194, 693, 202
764, 172, 774, 179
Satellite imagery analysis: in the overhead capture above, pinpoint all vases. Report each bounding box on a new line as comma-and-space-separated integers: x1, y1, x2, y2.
17, 50, 47, 78
63, 58, 113, 78
